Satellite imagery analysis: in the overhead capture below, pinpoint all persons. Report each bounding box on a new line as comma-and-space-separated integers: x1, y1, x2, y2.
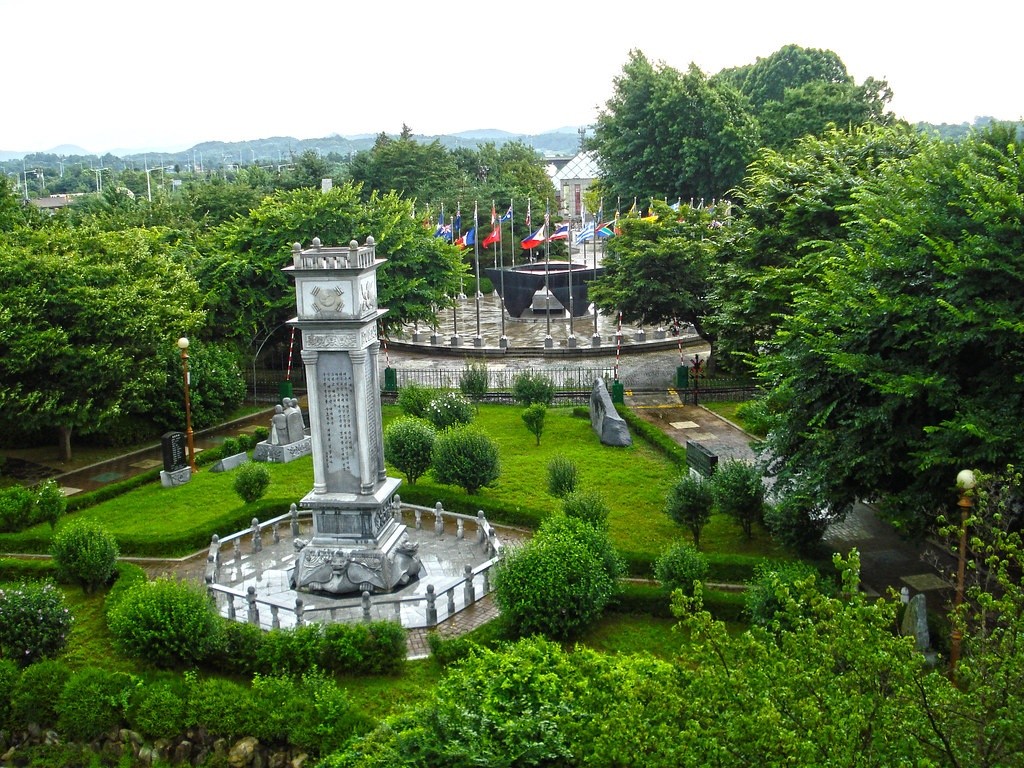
271, 397, 306, 446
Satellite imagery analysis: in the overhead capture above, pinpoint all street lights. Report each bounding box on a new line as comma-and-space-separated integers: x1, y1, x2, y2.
250, 150, 254, 163
239, 149, 243, 165
91, 167, 109, 195
87, 160, 93, 168
177, 337, 196, 473
948, 470, 975, 686
146, 166, 171, 218
75, 163, 81, 168
56, 158, 66, 176
186, 150, 203, 171
23, 158, 27, 172
25, 170, 37, 198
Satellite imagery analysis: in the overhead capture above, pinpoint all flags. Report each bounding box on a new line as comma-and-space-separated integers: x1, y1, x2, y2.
520, 223, 546, 250
455, 227, 475, 251
548, 222, 568, 242
576, 221, 594, 244
482, 225, 500, 249
410, 201, 722, 244
